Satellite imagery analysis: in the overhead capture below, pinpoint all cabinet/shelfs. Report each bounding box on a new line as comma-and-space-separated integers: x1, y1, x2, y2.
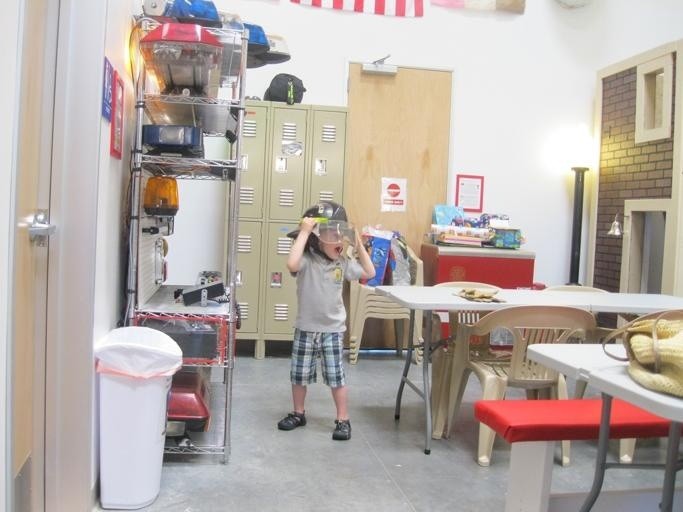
265, 102, 310, 221
226, 220, 265, 358
307, 105, 349, 217
226, 99, 271, 220
422, 238, 536, 362
261, 220, 301, 361
123, 13, 250, 466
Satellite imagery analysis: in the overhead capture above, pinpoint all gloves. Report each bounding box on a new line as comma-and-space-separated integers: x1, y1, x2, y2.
458, 287, 499, 302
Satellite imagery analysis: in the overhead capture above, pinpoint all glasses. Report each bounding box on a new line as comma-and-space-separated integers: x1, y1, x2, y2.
310, 219, 356, 247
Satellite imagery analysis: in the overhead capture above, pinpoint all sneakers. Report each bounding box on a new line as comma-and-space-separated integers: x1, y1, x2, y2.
277, 410, 306, 431
331, 418, 351, 440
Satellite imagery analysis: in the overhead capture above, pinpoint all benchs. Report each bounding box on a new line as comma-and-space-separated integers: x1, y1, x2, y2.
471, 397, 671, 512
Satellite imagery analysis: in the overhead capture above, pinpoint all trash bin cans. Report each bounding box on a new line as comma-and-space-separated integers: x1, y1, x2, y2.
96, 326, 183, 509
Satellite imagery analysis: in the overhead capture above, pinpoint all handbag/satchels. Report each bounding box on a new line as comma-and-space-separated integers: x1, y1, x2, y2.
601, 309, 682, 399
263, 74, 306, 105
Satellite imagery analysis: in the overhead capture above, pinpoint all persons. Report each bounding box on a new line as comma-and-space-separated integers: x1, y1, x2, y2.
277, 201, 376, 441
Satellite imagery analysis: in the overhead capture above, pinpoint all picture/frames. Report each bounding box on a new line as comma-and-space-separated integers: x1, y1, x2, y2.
454, 173, 484, 213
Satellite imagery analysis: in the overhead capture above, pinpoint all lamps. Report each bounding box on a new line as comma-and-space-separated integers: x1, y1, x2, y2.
565, 152, 590, 287
606, 209, 624, 238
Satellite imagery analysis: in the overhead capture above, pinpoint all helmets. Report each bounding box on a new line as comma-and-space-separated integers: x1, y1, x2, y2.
286, 198, 355, 248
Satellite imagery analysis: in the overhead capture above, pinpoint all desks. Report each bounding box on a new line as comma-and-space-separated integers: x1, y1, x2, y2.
526, 339, 682, 512
374, 285, 682, 455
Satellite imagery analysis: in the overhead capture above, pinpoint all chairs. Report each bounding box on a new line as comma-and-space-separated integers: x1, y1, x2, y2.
539, 284, 639, 399
442, 301, 598, 468
430, 281, 506, 440
347, 233, 425, 365
